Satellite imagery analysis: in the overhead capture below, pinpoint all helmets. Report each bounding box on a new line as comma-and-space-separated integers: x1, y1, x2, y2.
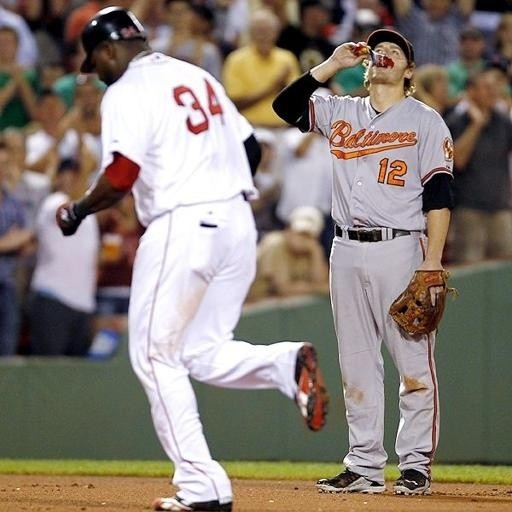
81, 7, 146, 75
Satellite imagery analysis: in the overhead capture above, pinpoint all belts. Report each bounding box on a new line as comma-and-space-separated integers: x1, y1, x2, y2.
336, 224, 410, 242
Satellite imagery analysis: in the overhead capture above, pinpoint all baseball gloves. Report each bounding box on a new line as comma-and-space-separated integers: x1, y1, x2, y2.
389, 269, 459, 338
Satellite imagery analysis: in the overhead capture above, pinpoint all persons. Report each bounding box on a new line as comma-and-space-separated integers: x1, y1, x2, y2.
129, 0, 380, 300
0, 1, 175, 357
342, 0, 512, 262
54, 5, 329, 511
272, 28, 453, 497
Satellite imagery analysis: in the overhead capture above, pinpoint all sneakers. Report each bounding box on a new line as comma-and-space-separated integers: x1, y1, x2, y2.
293, 343, 329, 430
394, 470, 433, 495
154, 496, 234, 511
315, 468, 387, 493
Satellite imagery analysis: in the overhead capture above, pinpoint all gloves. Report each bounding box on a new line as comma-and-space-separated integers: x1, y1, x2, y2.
55, 202, 80, 235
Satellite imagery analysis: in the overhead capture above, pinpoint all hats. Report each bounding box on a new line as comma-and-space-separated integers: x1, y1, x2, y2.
365, 28, 415, 66
